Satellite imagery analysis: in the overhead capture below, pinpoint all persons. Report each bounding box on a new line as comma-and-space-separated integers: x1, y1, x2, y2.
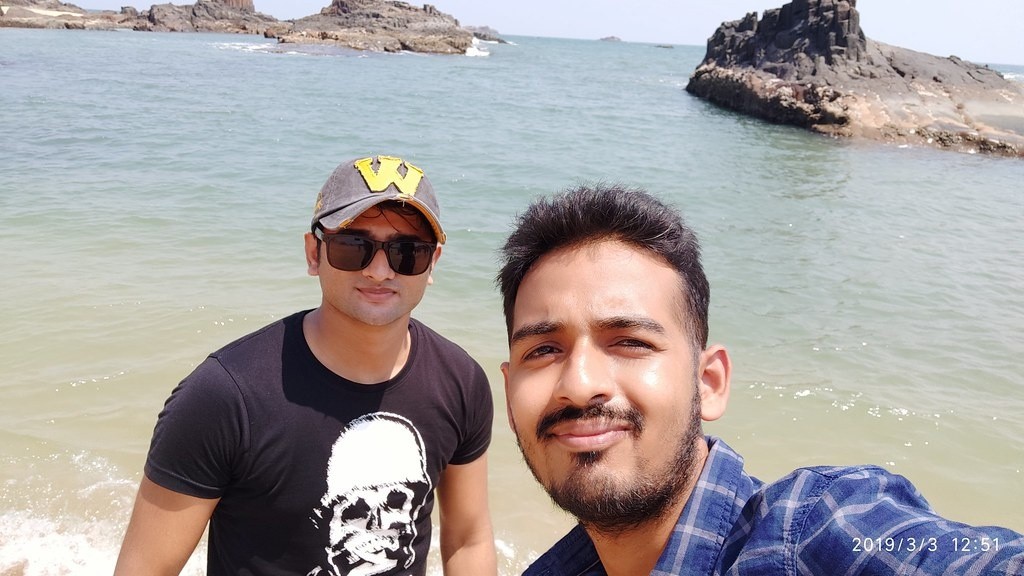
112, 154, 499, 576
498, 184, 1024, 576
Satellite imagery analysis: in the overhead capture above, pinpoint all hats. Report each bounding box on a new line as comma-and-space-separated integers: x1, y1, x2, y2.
311, 154, 447, 245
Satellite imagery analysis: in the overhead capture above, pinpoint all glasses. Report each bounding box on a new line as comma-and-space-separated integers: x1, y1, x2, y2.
314, 227, 436, 276
